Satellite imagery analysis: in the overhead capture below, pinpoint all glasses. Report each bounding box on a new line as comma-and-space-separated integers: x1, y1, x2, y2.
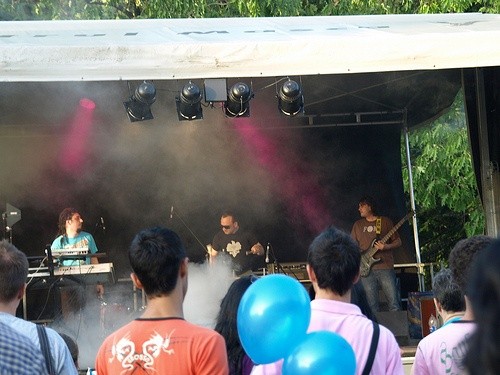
219, 224, 234, 229
249, 273, 258, 282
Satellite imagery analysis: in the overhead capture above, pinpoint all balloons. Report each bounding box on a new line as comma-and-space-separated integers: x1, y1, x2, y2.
281, 330, 357, 375
237, 274, 311, 366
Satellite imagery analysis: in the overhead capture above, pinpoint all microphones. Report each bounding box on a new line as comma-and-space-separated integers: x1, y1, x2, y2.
265, 246, 270, 262
168, 206, 173, 222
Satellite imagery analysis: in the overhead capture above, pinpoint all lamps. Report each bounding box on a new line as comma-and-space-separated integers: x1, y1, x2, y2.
275, 75, 307, 118
174, 79, 204, 121
122, 80, 157, 122
221, 77, 256, 118
202, 77, 229, 102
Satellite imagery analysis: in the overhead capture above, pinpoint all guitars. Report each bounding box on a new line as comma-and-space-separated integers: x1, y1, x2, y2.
360, 208, 419, 277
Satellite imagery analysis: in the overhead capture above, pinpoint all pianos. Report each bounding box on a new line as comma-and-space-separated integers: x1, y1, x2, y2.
27, 247, 118, 329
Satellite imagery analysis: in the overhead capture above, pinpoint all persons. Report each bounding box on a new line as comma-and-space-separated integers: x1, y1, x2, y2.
251, 226, 405, 375
0, 240, 80, 375
214, 275, 259, 375
206, 212, 265, 281
49, 206, 105, 331
95, 227, 230, 375
351, 191, 410, 346
412, 237, 500, 375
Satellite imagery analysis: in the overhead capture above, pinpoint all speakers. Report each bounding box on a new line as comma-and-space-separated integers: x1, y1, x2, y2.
374, 311, 409, 346
407, 292, 436, 339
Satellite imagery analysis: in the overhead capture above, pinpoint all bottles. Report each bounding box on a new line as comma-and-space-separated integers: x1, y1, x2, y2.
428, 313, 438, 333
